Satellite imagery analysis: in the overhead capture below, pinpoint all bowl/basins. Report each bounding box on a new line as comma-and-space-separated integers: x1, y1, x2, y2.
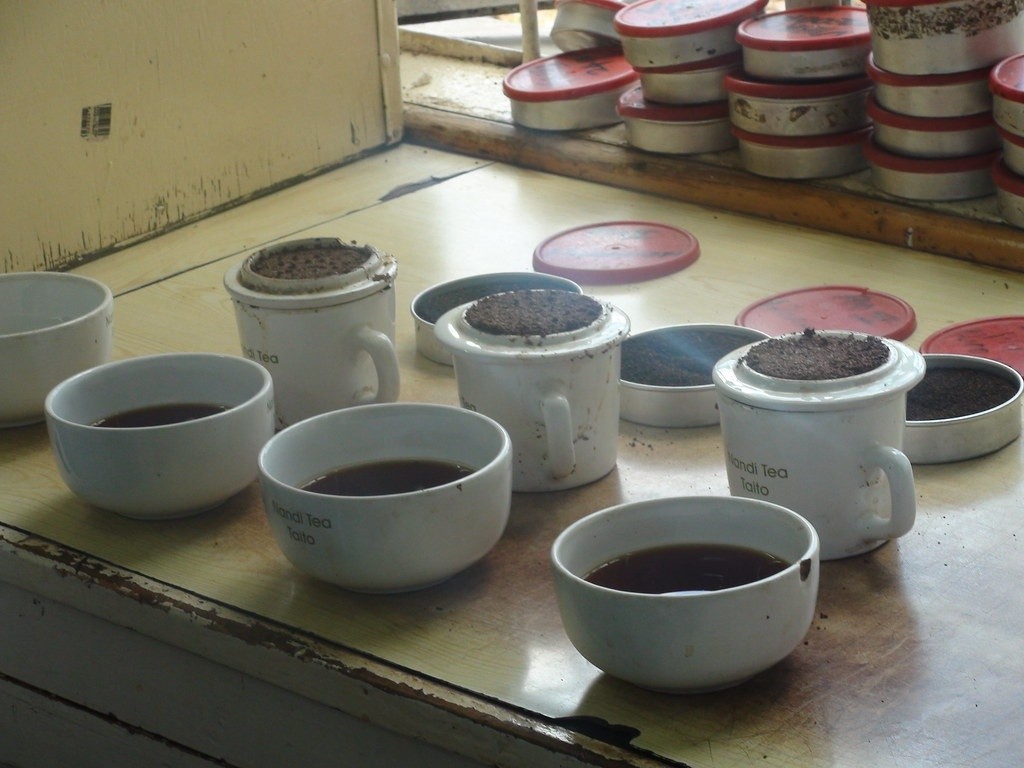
552, 492, 821, 696
0, 271, 114, 427
258, 401, 513, 594
46, 350, 276, 520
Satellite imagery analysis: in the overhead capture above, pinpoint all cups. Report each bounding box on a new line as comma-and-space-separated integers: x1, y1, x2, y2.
224, 236, 399, 431
434, 288, 633, 494
711, 329, 928, 562
